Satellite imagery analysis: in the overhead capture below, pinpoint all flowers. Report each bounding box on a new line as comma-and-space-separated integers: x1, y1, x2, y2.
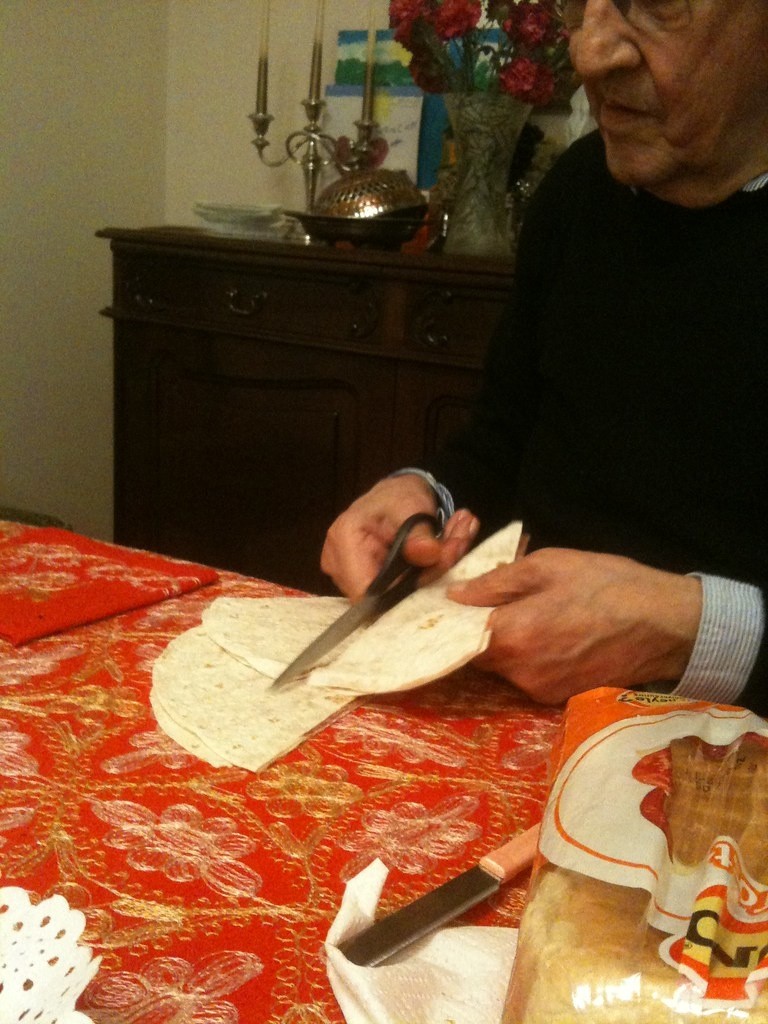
388, 0, 570, 108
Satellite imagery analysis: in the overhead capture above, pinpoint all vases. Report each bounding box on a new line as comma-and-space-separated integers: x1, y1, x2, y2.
441, 91, 535, 258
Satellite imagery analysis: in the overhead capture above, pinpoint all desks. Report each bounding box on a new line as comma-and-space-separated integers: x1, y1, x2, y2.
0, 521, 566, 1024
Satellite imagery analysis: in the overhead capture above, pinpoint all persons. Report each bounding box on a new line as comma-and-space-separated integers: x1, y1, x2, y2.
319, 0, 767, 719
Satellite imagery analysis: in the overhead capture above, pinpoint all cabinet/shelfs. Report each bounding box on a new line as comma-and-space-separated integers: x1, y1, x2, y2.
93, 224, 517, 597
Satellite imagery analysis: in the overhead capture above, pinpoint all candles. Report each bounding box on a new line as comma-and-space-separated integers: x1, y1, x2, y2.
259, 0, 270, 59
314, 0, 325, 44
366, 0, 377, 63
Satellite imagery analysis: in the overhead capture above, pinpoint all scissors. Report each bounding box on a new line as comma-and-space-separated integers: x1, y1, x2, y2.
269, 511, 444, 692
